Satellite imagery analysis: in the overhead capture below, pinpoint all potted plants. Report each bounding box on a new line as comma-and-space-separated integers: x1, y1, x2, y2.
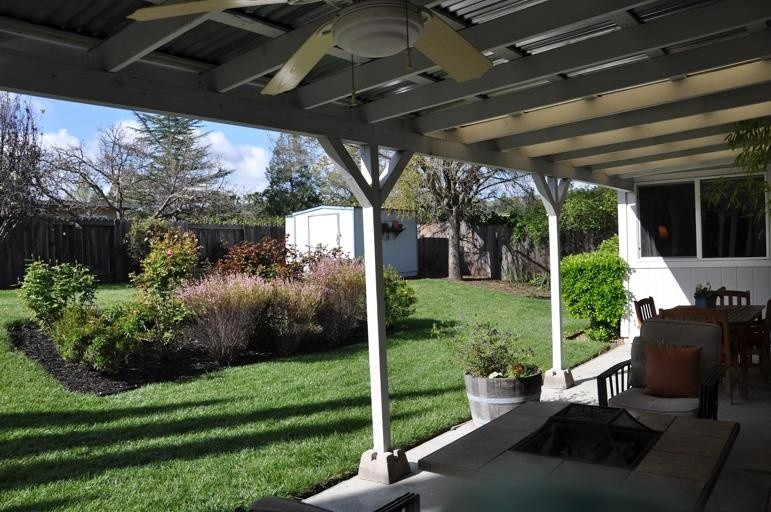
692, 284, 721, 307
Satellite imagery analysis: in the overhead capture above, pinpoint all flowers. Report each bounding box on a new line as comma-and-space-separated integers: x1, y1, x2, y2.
428, 307, 537, 379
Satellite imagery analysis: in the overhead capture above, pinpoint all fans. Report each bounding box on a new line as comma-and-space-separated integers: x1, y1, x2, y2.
127, 1, 493, 98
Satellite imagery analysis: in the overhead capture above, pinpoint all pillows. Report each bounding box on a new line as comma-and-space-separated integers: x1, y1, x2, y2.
628, 333, 706, 398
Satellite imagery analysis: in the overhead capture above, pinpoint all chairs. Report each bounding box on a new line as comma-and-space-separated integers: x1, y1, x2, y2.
597, 316, 726, 422
633, 289, 771, 404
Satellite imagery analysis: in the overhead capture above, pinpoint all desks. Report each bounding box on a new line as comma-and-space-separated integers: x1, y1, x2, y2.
418, 398, 740, 509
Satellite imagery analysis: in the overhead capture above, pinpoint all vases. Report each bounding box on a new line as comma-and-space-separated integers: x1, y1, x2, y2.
464, 371, 544, 427
